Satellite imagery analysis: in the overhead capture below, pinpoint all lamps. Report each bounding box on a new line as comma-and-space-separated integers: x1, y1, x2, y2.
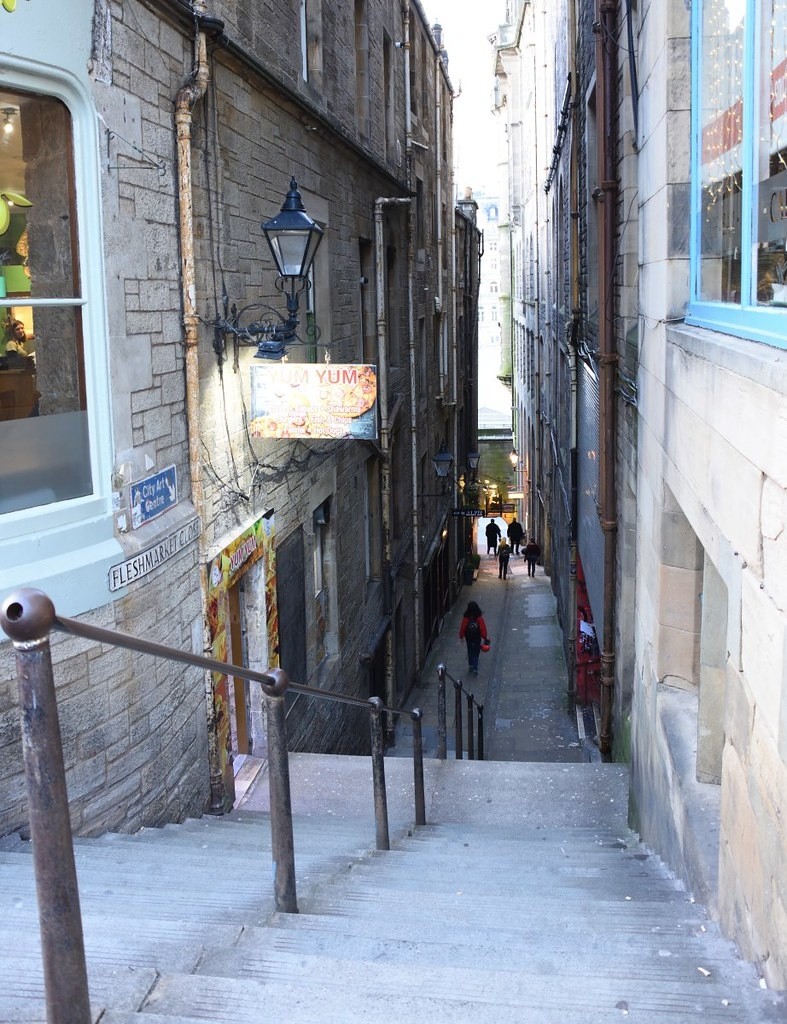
419, 443, 452, 498
508, 450, 528, 472
451, 448, 479, 482
212, 177, 322, 361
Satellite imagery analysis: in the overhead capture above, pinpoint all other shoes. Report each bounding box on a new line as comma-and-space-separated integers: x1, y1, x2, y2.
528, 572, 534, 577
470, 667, 478, 676
498, 575, 507, 580
515, 551, 519, 555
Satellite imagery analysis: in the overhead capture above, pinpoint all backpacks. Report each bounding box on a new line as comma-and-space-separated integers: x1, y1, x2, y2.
465, 617, 481, 642
499, 545, 509, 563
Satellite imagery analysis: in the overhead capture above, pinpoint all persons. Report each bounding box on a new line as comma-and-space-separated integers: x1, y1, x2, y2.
525, 538, 541, 577
486, 519, 502, 555
459, 601, 487, 676
495, 537, 510, 580
507, 517, 523, 554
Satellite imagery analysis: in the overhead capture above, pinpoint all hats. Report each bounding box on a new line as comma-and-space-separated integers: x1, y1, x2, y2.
480, 644, 490, 652
